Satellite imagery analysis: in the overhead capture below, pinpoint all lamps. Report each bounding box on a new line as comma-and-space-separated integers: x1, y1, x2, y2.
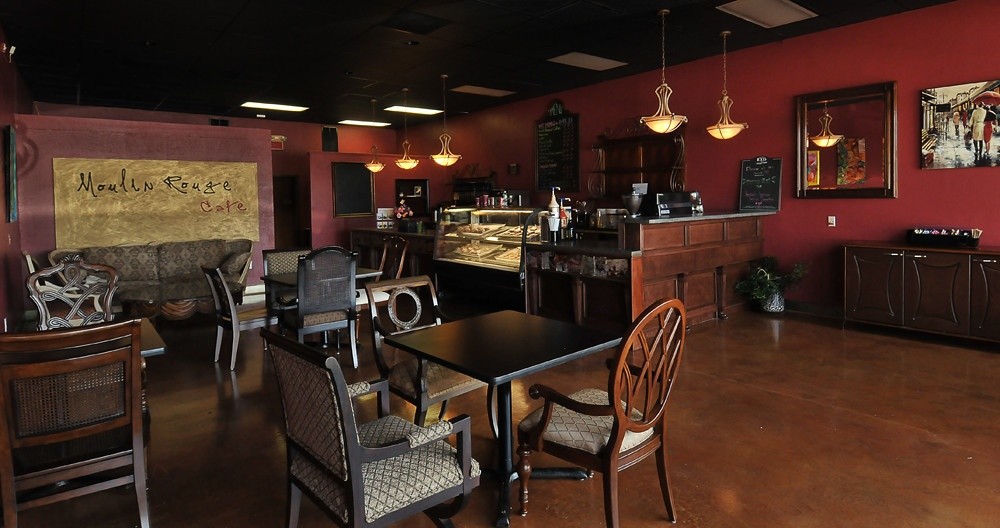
8, 46, 17, 64
706, 31, 749, 141
810, 101, 844, 148
431, 75, 462, 167
396, 88, 420, 170
640, 9, 688, 133
364, 99, 387, 173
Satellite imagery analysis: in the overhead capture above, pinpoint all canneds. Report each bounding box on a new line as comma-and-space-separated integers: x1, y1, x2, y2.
475, 194, 495, 207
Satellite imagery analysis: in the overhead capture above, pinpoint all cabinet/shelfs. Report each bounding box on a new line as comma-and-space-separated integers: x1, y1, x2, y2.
346, 207, 764, 352
844, 240, 1000, 345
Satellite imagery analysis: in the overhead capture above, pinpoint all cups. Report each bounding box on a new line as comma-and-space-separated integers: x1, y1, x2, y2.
475, 194, 494, 206
416, 223, 422, 232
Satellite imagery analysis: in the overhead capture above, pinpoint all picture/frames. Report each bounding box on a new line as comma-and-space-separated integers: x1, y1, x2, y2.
807, 151, 820, 186
332, 162, 376, 219
5, 124, 18, 224
739, 158, 783, 212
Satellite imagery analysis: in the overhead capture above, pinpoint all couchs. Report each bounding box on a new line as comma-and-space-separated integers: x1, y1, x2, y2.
47, 239, 254, 321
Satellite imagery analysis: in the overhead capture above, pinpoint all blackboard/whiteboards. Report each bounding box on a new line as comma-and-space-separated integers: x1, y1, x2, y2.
739, 156, 785, 213
535, 99, 580, 192
332, 161, 376, 218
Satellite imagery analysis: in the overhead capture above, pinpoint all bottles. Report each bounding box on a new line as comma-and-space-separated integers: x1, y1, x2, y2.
494, 190, 508, 208
567, 217, 574, 238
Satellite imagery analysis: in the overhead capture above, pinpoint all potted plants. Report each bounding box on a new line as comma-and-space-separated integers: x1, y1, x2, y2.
733, 256, 809, 313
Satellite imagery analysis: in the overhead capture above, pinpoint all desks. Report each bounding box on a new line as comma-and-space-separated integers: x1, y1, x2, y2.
384, 310, 624, 528
260, 267, 384, 350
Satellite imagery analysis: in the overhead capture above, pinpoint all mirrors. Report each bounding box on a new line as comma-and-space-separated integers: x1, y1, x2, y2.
795, 81, 899, 200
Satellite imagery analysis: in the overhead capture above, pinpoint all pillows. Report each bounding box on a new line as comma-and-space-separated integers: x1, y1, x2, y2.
219, 251, 251, 274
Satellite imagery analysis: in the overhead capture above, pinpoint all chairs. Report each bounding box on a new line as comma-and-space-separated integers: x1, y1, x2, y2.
262, 247, 311, 301
259, 328, 482, 528
139, 316, 168, 490
26, 253, 118, 330
200, 264, 278, 370
0, 318, 150, 528
516, 296, 688, 528
356, 237, 409, 343
279, 245, 360, 371
363, 274, 498, 440
